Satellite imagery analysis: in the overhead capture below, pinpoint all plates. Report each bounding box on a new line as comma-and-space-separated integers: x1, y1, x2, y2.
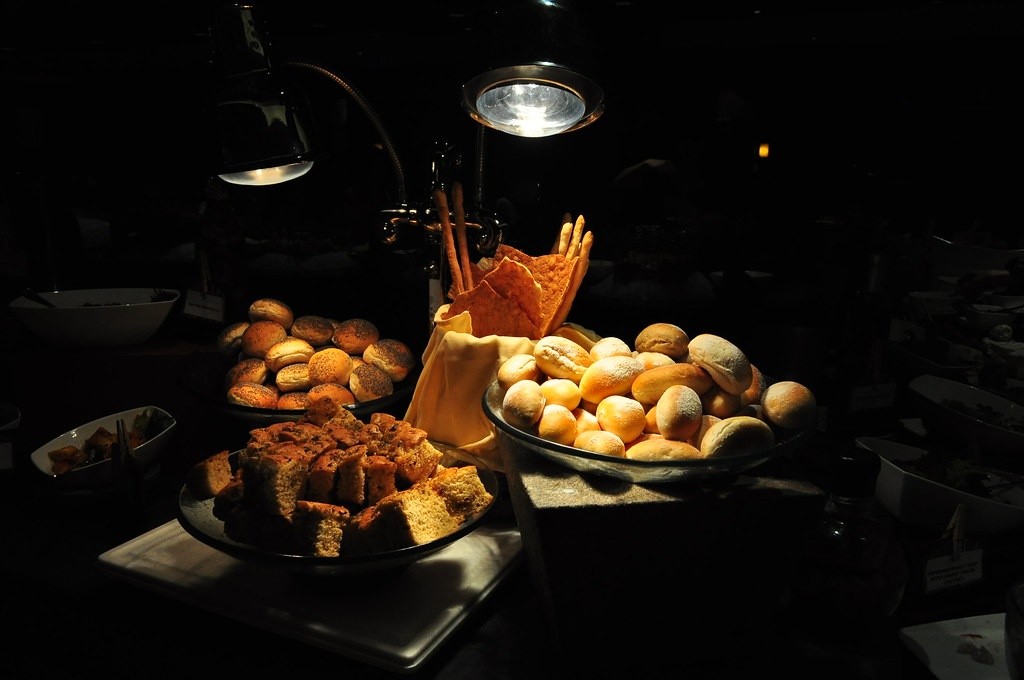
31, 407, 176, 479
481, 379, 814, 480
216, 381, 417, 427
173, 439, 498, 579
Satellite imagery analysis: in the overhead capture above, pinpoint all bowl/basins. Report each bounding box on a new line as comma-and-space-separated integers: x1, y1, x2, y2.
888, 319, 983, 369
856, 437, 1024, 534
967, 305, 1020, 330
909, 374, 1023, 453
11, 287, 179, 340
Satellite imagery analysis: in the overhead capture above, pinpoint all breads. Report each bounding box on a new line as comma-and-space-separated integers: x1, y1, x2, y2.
186, 186, 815, 560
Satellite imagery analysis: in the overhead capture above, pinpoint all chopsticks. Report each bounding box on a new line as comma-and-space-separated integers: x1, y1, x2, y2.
114, 418, 135, 466
